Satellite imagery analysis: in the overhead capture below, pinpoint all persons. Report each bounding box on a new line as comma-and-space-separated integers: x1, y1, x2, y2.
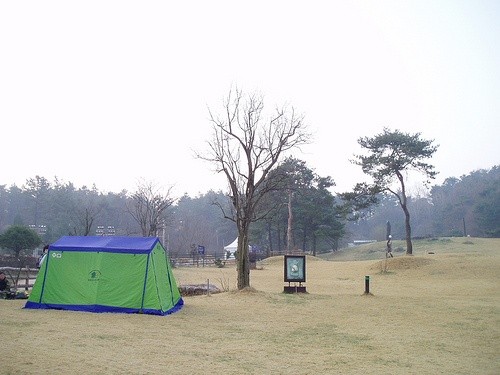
0, 271, 10, 300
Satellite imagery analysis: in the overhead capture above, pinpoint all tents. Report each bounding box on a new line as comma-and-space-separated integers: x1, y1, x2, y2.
21, 235, 184, 316
224, 237, 252, 260
